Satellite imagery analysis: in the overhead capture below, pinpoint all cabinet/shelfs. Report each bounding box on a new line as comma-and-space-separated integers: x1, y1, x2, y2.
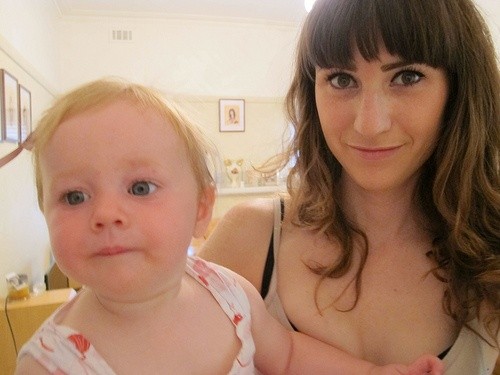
0, 289, 77, 375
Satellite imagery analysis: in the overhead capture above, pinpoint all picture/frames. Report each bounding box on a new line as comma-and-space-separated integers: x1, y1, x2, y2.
219, 99, 245, 132
1, 69, 19, 143
19, 84, 32, 151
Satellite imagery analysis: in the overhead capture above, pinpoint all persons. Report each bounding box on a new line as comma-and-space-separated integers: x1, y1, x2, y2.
13, 79, 446, 375
193, 0, 500, 375
